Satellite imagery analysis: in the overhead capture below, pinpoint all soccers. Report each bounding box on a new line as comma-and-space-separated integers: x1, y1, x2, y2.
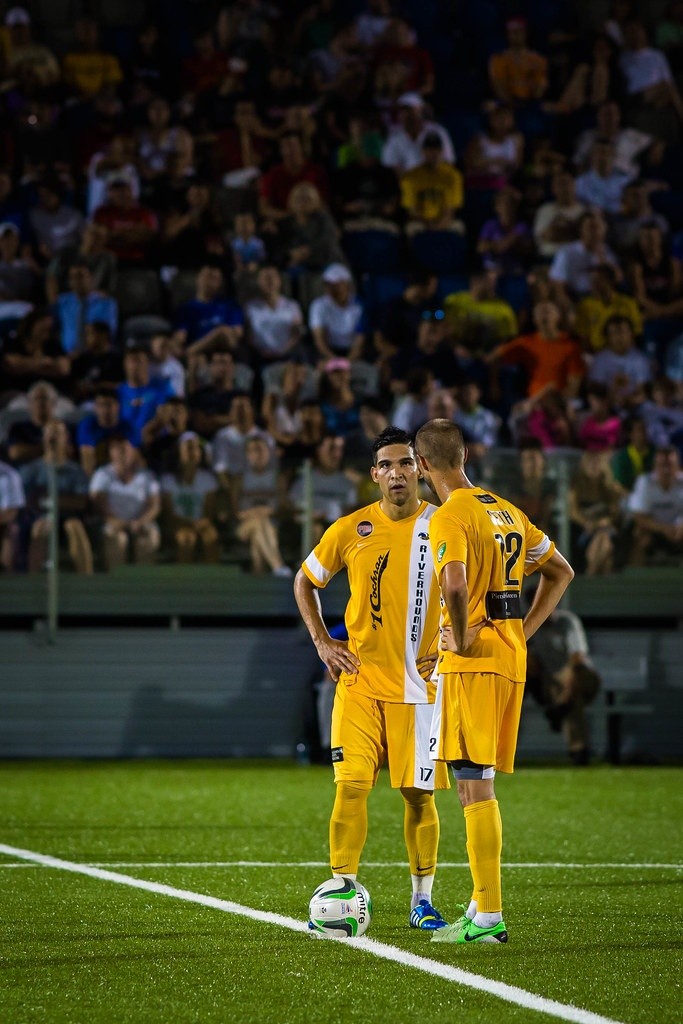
309, 877, 373, 938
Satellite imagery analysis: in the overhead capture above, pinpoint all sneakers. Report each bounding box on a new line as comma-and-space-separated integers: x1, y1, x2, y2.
309, 921, 317, 930
431, 919, 509, 943
410, 900, 451, 929
434, 914, 473, 937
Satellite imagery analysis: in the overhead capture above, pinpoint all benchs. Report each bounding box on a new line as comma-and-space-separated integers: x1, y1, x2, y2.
524, 631, 654, 765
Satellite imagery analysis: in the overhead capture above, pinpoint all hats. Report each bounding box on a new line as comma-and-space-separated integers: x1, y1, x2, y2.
398, 92, 424, 110
322, 262, 354, 284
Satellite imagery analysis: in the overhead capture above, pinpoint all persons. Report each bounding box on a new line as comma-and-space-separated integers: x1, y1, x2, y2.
414, 416, 576, 941
294, 426, 452, 926
0, 1, 683, 768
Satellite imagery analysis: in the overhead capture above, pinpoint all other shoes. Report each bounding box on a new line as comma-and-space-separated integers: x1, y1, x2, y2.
275, 564, 294, 579
